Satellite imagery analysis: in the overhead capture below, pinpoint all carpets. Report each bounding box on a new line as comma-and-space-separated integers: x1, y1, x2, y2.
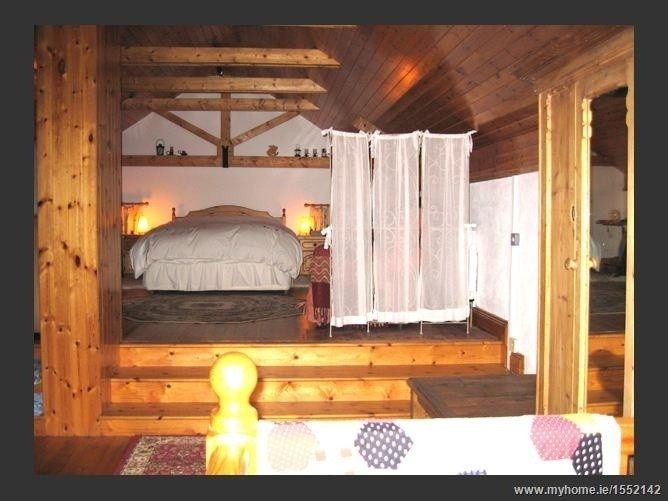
120, 290, 309, 325
106, 432, 207, 475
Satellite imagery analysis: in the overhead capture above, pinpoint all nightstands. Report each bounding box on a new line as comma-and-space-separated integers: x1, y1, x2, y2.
298, 231, 332, 277
122, 234, 145, 275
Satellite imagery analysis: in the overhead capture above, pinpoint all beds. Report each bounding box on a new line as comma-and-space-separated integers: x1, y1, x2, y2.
141, 204, 298, 297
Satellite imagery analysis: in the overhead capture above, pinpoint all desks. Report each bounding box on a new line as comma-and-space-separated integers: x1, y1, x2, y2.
595, 218, 629, 277
406, 374, 539, 418
